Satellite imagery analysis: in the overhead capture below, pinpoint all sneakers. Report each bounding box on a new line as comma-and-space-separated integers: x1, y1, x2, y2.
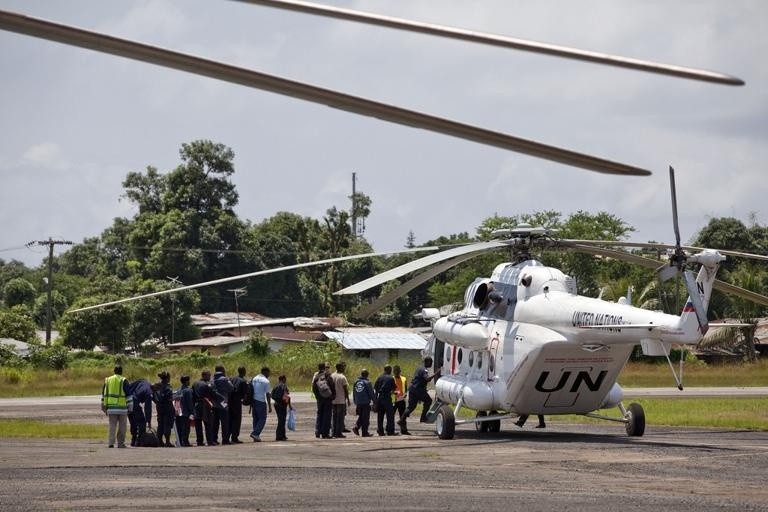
160, 441, 219, 447
222, 439, 241, 444
379, 431, 411, 436
362, 433, 373, 437
316, 429, 350, 438
353, 427, 359, 435
250, 433, 261, 441
398, 421, 406, 430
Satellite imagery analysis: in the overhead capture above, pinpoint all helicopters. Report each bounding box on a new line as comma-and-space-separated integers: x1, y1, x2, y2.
62, 221, 768, 441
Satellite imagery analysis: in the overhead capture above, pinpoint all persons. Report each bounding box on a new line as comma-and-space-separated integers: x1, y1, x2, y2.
397, 356, 440, 425
312, 360, 410, 437
101, 365, 297, 448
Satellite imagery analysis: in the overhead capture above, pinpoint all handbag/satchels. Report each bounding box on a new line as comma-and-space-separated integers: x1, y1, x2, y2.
140, 433, 158, 447
242, 381, 254, 406
317, 376, 332, 398
